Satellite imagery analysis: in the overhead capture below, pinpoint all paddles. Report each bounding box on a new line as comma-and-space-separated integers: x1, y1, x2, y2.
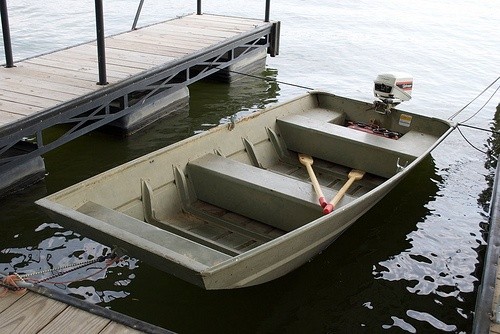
298, 153, 328, 208
323, 169, 365, 214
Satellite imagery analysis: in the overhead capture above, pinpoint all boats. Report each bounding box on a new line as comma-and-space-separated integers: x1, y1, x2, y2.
35, 71, 457, 290
